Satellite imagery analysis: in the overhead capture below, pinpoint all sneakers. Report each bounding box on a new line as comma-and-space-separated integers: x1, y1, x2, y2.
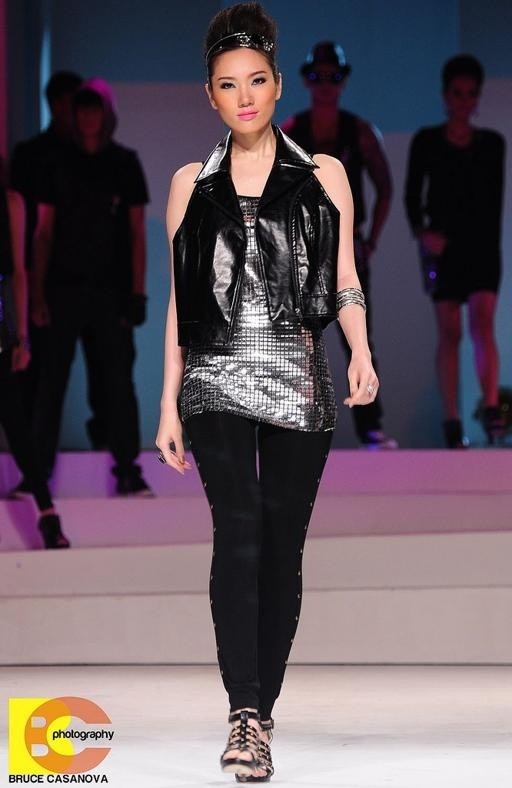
362, 429, 399, 449
17, 480, 32, 491
113, 464, 147, 491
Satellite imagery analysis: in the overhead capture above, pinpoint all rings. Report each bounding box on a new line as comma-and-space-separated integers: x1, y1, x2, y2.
158, 452, 167, 465
367, 384, 375, 394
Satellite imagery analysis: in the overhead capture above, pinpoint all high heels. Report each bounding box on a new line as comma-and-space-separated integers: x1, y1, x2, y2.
40, 515, 69, 548
484, 407, 510, 443
444, 420, 463, 445
220, 711, 274, 782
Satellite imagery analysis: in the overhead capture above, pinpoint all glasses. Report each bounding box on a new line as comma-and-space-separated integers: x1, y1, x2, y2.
310, 71, 345, 84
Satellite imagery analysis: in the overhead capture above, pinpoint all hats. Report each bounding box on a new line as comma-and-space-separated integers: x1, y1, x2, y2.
300, 41, 352, 73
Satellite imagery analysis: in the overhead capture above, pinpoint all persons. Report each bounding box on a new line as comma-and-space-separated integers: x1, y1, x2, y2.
148, 0, 383, 788
2, 68, 138, 457
397, 50, 512, 451
3, 190, 75, 553
269, 37, 406, 452
4, 74, 158, 504
